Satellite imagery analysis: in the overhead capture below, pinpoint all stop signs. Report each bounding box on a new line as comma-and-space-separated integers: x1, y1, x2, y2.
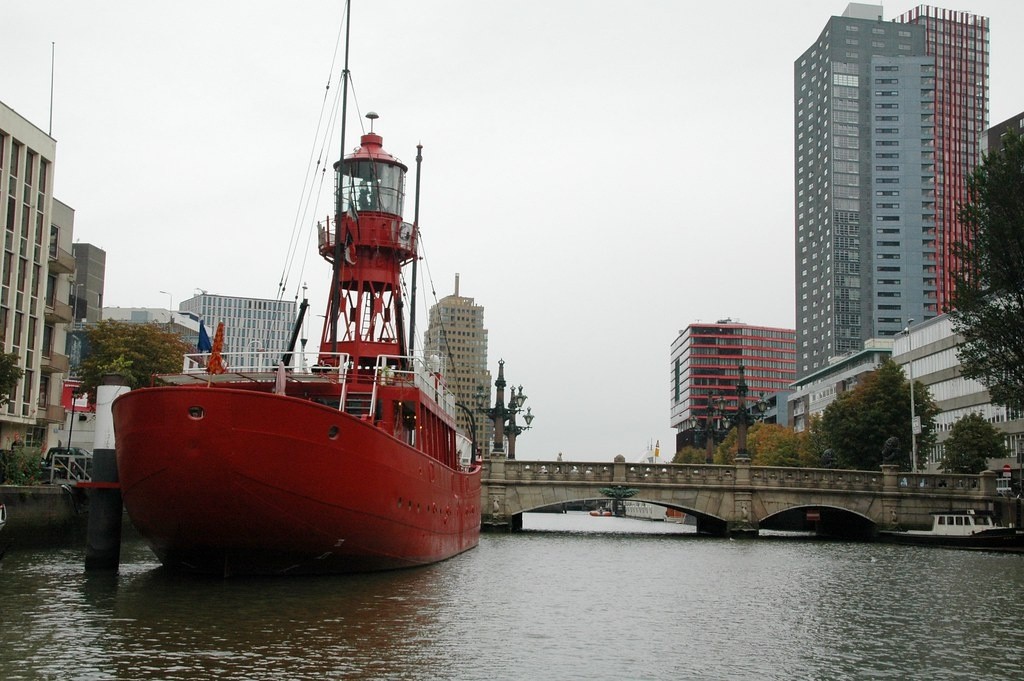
1003, 465, 1011, 472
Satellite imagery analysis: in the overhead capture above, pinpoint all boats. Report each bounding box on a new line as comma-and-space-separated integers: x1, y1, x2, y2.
878, 509, 1024, 553
590, 511, 611, 516
113, 0, 484, 575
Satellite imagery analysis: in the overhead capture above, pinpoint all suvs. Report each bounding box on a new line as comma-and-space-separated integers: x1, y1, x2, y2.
46, 446, 93, 478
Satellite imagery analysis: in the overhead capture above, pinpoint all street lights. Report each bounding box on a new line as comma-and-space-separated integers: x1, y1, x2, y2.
502, 386, 535, 459
901, 313, 917, 473
64, 387, 80, 478
473, 358, 527, 452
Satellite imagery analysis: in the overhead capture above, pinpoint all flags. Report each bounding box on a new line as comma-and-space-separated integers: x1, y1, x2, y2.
347, 190, 359, 222
344, 223, 358, 265
